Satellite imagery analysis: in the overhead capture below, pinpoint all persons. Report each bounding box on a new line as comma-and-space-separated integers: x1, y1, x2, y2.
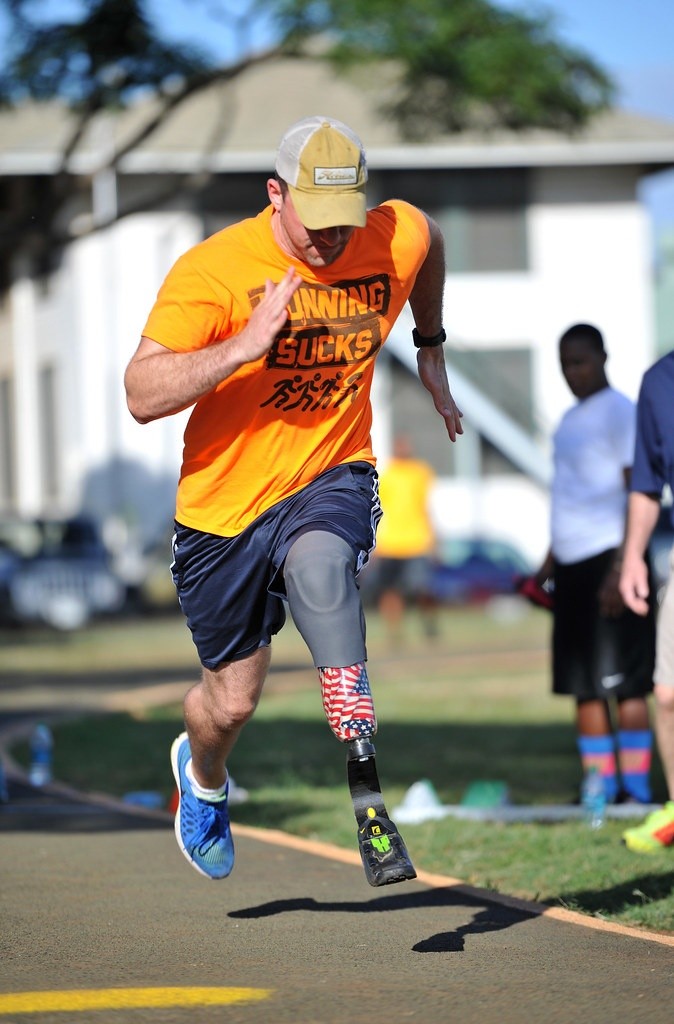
371, 433, 447, 651
619, 350, 674, 854
535, 323, 659, 808
123, 115, 464, 886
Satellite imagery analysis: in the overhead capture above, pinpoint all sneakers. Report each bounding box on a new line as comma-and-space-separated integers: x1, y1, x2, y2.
357, 816, 417, 887
169, 731, 236, 880
622, 801, 674, 854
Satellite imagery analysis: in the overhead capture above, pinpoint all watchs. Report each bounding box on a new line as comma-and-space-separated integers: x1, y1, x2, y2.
412, 327, 447, 348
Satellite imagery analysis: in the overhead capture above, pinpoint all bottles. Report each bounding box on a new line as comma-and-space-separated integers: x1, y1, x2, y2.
509, 573, 553, 610
580, 764, 605, 831
27, 721, 55, 788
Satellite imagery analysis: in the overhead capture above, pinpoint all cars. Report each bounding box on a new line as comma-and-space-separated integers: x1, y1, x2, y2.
410, 529, 532, 597
1, 511, 134, 627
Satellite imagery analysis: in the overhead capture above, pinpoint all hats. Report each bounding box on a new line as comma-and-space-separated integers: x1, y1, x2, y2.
274, 115, 370, 231
